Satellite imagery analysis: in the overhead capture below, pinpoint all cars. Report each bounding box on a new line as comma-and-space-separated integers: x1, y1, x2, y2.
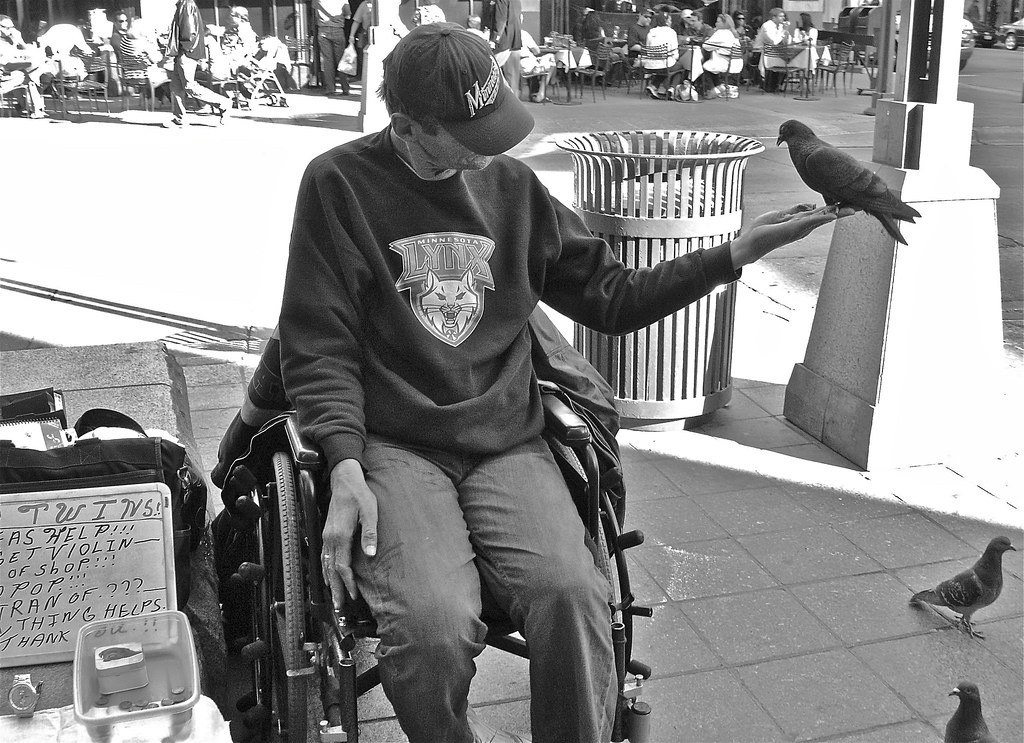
963, 13, 998, 49
892, 10, 975, 72
995, 17, 1024, 50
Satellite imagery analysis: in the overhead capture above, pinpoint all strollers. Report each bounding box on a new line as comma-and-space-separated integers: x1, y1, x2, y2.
232, 35, 290, 109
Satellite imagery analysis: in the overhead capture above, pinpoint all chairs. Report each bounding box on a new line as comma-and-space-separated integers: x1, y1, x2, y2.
0, 35, 315, 120
514, 35, 855, 103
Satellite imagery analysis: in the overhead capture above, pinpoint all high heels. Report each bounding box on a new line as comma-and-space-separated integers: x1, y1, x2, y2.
281, 97, 287, 107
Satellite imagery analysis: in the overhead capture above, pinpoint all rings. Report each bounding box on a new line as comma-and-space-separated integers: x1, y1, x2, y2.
325, 554, 331, 559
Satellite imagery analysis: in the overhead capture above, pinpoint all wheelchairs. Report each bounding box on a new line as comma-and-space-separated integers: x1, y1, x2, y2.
234, 305, 652, 742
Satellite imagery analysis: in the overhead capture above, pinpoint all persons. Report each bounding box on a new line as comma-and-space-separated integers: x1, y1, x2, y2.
0, 0, 257, 128
311, 0, 372, 96
733, 8, 818, 94
569, 7, 742, 102
411, 0, 556, 104
280, 21, 858, 743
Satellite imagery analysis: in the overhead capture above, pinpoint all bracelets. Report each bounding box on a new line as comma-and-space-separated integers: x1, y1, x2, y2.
349, 36, 355, 39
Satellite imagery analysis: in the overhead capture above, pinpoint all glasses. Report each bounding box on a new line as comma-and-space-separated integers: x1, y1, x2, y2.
642, 14, 652, 19
0, 24, 15, 30
118, 19, 129, 23
737, 17, 746, 21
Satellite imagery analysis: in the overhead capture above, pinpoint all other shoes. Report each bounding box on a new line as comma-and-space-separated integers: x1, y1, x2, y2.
30, 110, 49, 119
220, 98, 232, 125
164, 118, 190, 129
703, 85, 726, 99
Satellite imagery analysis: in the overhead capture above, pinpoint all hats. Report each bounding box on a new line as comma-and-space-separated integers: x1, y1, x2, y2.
583, 8, 595, 14
639, 8, 655, 16
383, 21, 535, 156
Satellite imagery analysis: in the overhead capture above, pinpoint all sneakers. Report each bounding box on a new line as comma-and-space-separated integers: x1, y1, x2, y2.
645, 86, 660, 99
667, 87, 675, 101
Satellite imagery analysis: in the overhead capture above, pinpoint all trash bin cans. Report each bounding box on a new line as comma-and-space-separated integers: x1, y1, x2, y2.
555, 129, 767, 435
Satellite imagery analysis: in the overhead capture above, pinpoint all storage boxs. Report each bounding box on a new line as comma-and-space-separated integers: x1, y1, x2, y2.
95, 642, 149, 694
73, 610, 201, 743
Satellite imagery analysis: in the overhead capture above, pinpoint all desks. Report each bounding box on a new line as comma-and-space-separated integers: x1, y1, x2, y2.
679, 44, 704, 82
607, 39, 629, 83
538, 46, 592, 99
764, 45, 832, 94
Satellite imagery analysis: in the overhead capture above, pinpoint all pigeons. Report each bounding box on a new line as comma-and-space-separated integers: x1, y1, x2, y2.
910, 536, 1016, 640
944, 681, 999, 743
777, 120, 922, 247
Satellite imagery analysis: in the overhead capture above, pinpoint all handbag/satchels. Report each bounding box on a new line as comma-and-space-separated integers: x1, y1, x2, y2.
337, 42, 357, 75
0, 437, 209, 613
